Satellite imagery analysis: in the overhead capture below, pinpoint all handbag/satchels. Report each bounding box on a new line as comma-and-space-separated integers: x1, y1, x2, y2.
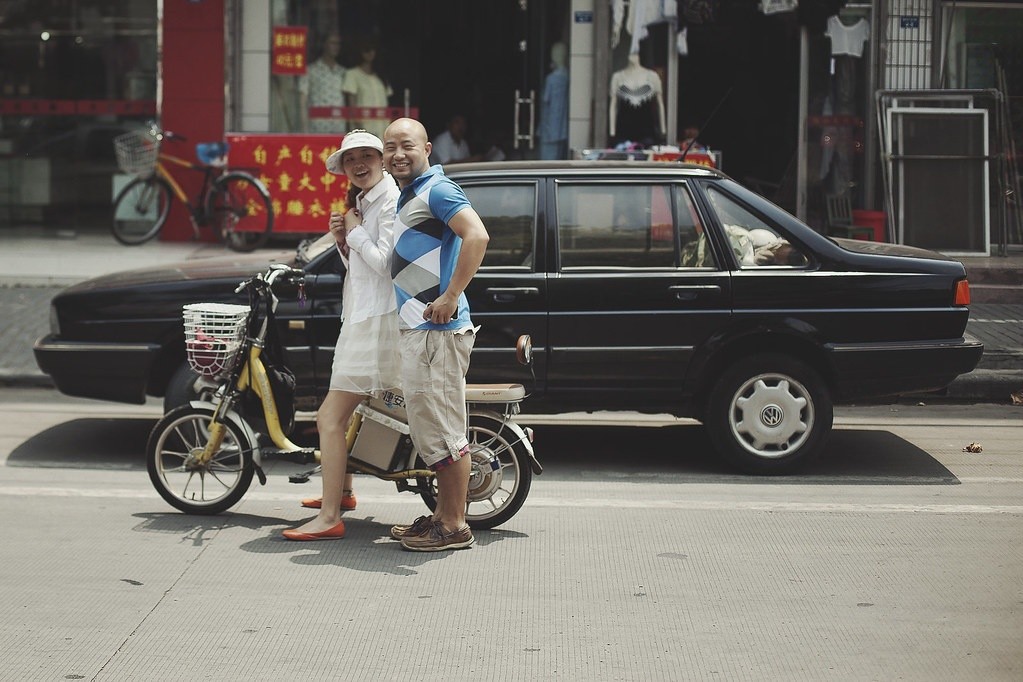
230, 278, 297, 438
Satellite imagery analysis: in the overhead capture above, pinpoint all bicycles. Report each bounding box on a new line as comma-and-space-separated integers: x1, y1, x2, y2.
107, 117, 275, 254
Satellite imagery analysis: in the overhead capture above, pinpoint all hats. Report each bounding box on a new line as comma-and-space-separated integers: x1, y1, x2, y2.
325, 129, 384, 175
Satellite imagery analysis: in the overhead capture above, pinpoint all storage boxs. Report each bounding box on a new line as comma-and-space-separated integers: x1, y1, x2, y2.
849, 206, 887, 242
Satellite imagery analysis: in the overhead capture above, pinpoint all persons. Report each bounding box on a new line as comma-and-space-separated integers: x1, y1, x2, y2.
610, 56, 665, 150
536, 43, 570, 160
283, 130, 402, 540
432, 118, 485, 166
384, 118, 490, 552
681, 120, 704, 149
300, 32, 390, 140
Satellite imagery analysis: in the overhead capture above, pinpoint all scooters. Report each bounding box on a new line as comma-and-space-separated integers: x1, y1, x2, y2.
143, 262, 546, 530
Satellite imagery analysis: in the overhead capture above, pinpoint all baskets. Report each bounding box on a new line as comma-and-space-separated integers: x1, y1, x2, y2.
182, 302, 251, 376
114, 131, 159, 178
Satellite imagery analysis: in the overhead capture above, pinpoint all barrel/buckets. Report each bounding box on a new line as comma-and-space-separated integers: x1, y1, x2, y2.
851, 208, 887, 242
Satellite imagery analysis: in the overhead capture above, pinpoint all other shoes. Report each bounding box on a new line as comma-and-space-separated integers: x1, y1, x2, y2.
282, 519, 345, 540
301, 496, 356, 510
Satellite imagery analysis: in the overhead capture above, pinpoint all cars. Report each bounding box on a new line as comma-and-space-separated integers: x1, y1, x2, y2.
32, 161, 985, 477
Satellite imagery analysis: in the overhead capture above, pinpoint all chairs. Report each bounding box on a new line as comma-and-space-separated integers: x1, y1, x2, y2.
823, 189, 875, 241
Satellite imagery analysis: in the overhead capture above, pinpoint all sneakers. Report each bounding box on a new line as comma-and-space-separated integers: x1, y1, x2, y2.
401, 514, 475, 551
390, 515, 434, 538
